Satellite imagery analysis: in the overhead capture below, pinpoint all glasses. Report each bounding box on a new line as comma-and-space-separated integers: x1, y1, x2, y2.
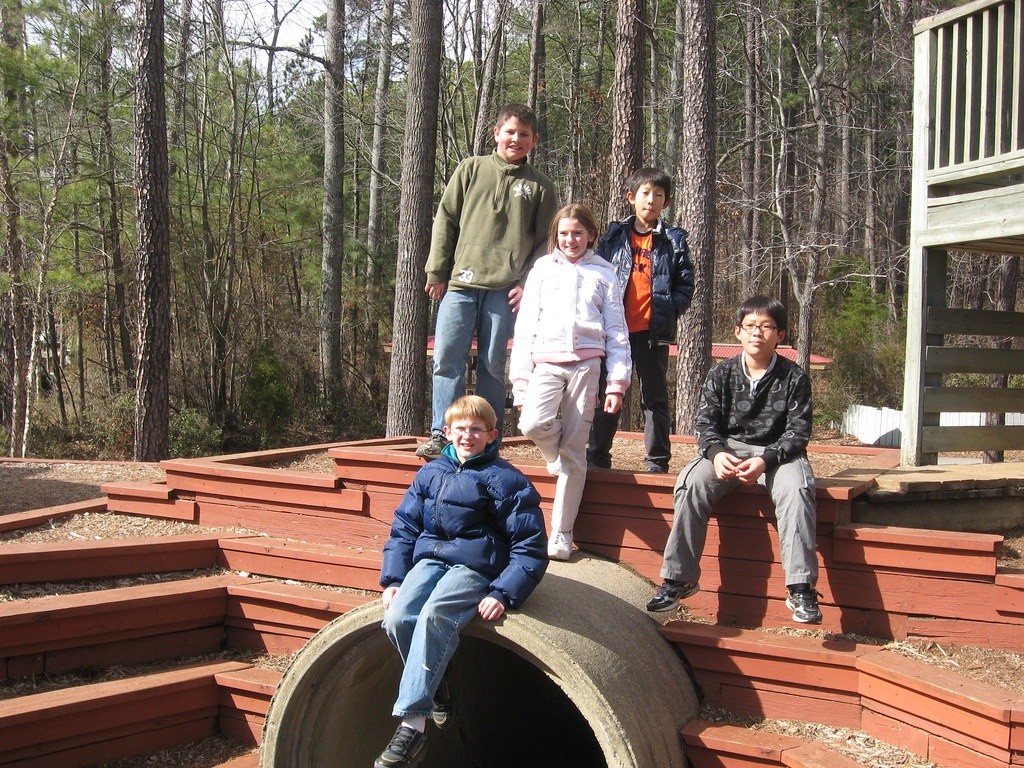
736, 322, 780, 334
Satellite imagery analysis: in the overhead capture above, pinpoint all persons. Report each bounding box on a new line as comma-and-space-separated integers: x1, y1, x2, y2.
415, 104, 558, 460
374, 395, 550, 768
587, 168, 695, 473
646, 295, 823, 625
509, 204, 633, 561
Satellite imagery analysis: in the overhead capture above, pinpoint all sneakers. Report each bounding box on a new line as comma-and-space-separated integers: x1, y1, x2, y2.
786, 583, 823, 626
415, 433, 452, 459
646, 578, 700, 612
374, 721, 431, 768
547, 452, 562, 476
432, 683, 456, 730
546, 529, 574, 560
